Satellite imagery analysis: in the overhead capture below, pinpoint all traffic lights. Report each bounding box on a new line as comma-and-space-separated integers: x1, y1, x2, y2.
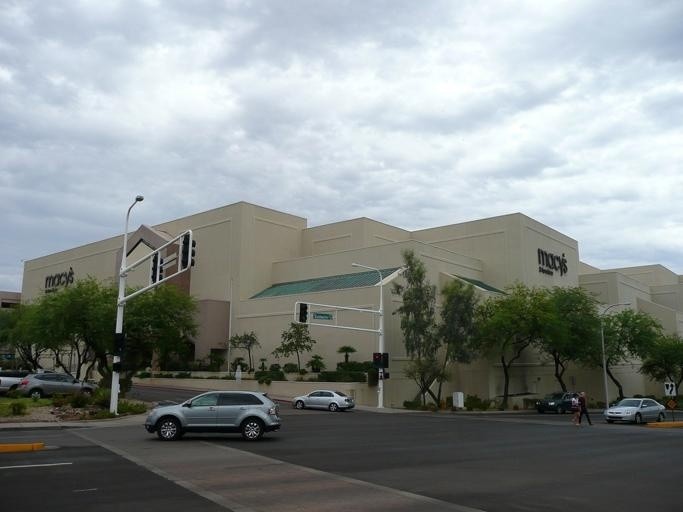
374, 353, 381, 369
181, 234, 196, 267
300, 304, 308, 322
151, 255, 163, 281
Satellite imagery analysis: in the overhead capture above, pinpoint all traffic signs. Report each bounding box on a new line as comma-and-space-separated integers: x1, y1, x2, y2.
313, 312, 333, 319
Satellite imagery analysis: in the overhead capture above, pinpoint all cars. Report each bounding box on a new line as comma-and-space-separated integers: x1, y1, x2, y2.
292, 390, 354, 412
1, 369, 96, 399
604, 398, 666, 424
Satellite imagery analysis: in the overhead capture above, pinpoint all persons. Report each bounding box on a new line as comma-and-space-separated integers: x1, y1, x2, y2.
571, 393, 581, 422
579, 392, 594, 425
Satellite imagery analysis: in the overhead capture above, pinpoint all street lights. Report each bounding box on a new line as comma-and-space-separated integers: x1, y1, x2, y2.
600, 302, 630, 408
109, 195, 143, 412
351, 263, 384, 406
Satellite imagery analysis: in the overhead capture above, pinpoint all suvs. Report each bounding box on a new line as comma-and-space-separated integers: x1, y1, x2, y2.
536, 392, 581, 414
145, 391, 281, 441
